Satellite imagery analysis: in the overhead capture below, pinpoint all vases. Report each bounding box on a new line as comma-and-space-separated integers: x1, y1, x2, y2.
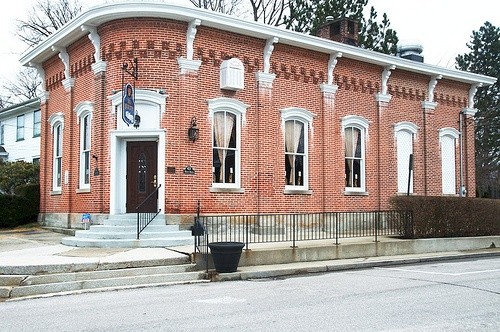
208, 241, 244, 273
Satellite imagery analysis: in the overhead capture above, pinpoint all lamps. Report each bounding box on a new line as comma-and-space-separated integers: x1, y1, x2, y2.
188, 117, 200, 143
